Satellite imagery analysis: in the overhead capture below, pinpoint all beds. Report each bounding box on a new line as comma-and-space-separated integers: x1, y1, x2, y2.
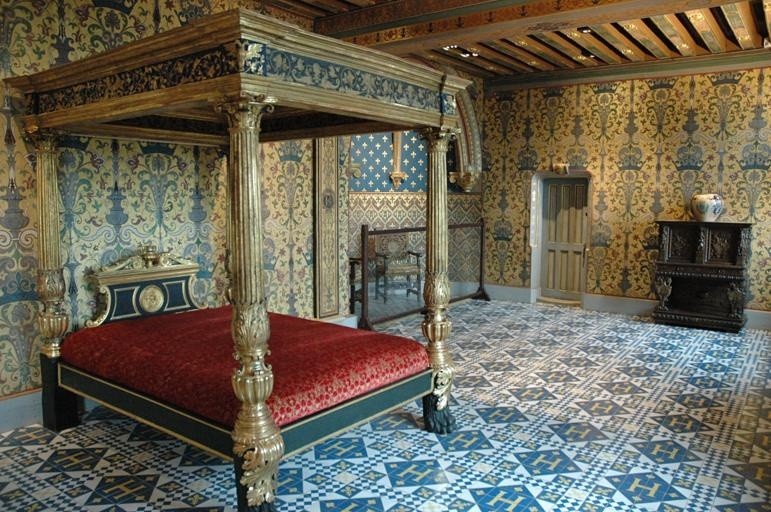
1, 6, 474, 512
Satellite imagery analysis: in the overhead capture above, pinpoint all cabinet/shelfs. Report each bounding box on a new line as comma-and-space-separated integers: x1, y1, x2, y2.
650, 219, 753, 334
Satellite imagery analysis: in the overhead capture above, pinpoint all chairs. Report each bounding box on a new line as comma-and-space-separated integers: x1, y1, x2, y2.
348, 256, 378, 314
373, 226, 423, 304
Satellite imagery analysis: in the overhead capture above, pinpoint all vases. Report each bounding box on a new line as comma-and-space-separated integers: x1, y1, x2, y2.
689, 193, 724, 223
